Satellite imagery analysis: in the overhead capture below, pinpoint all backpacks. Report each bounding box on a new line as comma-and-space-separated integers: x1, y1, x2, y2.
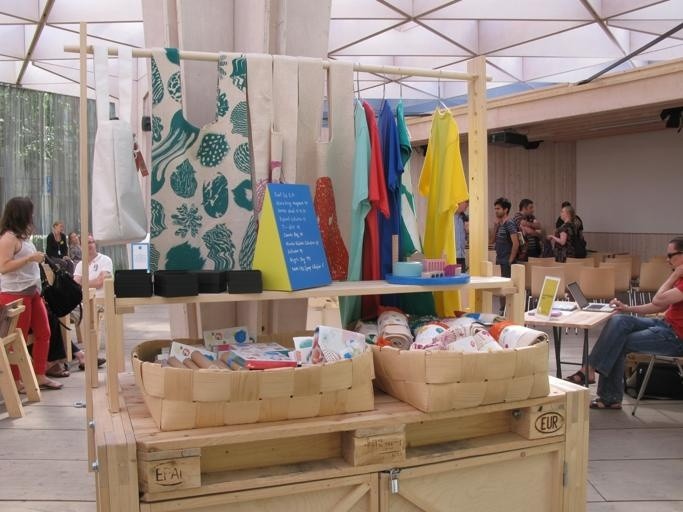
566, 227, 586, 258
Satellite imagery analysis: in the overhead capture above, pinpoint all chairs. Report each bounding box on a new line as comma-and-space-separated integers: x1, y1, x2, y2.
461, 247, 672, 320
626, 351, 683, 416
0, 298, 41, 418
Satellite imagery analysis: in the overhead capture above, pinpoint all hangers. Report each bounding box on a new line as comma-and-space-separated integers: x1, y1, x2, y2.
379, 64, 389, 115
436, 69, 451, 115
354, 63, 365, 107
396, 67, 404, 106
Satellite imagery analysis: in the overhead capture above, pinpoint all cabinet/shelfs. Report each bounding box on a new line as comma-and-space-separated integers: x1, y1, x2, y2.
93, 261, 589, 512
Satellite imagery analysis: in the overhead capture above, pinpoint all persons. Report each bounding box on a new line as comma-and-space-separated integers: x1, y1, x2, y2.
513, 199, 547, 262
545, 206, 580, 263
0, 197, 65, 395
455, 200, 468, 273
494, 197, 519, 316
562, 237, 683, 409
39, 263, 106, 378
68, 232, 82, 267
73, 235, 117, 307
556, 202, 583, 240
45, 220, 68, 259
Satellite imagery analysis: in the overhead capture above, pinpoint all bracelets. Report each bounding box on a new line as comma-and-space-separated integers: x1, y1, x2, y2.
625, 305, 628, 312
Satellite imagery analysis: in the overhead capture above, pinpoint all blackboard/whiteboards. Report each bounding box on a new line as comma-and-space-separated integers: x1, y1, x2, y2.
576, 128, 683, 235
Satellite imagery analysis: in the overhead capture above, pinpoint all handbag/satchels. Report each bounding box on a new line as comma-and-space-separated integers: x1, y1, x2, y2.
89, 119, 150, 248
40, 270, 82, 318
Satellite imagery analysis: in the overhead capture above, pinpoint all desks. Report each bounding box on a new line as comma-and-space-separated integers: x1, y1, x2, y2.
523, 301, 627, 379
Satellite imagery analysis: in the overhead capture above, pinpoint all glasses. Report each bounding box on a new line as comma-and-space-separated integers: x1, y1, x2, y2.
667, 252, 680, 259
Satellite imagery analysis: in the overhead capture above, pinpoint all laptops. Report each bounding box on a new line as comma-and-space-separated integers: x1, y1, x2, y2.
567, 281, 616, 312
524, 276, 561, 321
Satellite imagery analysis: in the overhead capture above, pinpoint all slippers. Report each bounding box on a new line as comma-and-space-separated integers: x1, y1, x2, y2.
564, 371, 595, 385
39, 382, 64, 390
78, 358, 106, 371
590, 399, 622, 409
46, 363, 70, 377
